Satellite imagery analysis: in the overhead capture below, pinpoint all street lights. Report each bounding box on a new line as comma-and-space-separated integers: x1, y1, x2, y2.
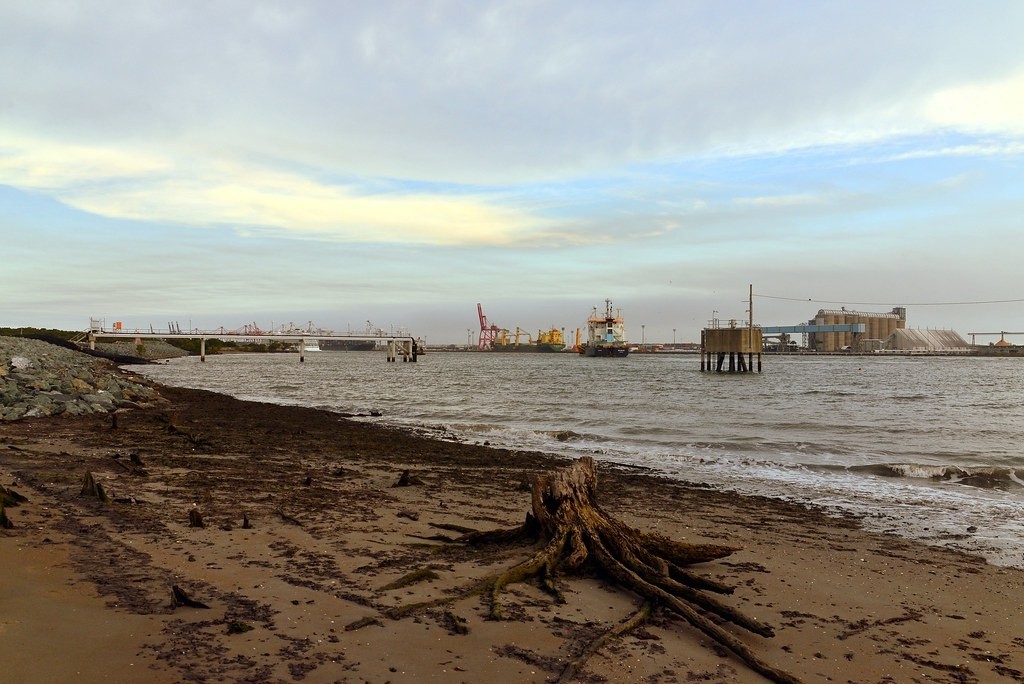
641, 324, 645, 346
673, 328, 676, 350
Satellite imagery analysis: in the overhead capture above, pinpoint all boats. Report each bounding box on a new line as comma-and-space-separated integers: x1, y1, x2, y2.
491, 329, 566, 352
398, 348, 426, 355
585, 299, 629, 358
